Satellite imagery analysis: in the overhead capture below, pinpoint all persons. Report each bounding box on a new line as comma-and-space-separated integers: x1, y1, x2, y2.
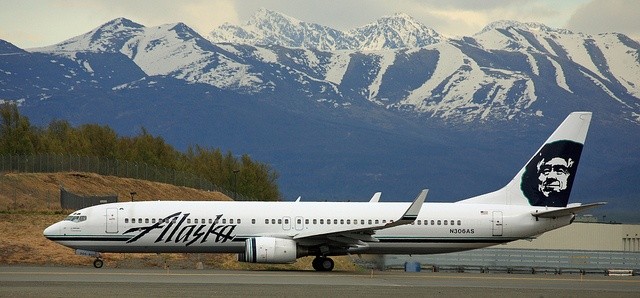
535, 156, 574, 198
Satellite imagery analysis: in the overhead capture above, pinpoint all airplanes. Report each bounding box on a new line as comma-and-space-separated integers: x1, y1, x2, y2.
43, 111, 607, 272
